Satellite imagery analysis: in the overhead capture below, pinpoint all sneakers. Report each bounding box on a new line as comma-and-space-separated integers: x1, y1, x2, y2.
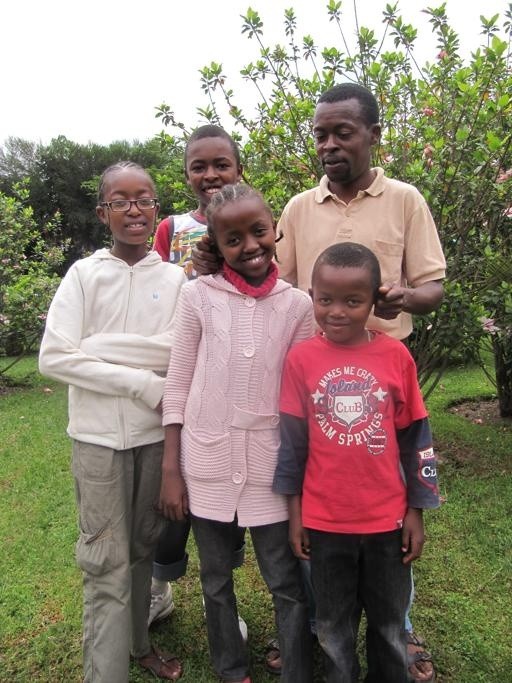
147, 581, 175, 628
203, 596, 247, 643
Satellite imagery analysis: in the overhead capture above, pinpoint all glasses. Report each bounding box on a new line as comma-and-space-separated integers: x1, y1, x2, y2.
100, 198, 156, 213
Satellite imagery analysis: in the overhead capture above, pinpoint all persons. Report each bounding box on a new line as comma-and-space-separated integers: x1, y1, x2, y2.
38, 160, 188, 683
270, 243, 439, 682
147, 125, 249, 647
159, 185, 315, 683
188, 81, 445, 682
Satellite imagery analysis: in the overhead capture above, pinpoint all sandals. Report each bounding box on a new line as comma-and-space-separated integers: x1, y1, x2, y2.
265, 637, 283, 675
404, 633, 434, 681
138, 646, 183, 680
